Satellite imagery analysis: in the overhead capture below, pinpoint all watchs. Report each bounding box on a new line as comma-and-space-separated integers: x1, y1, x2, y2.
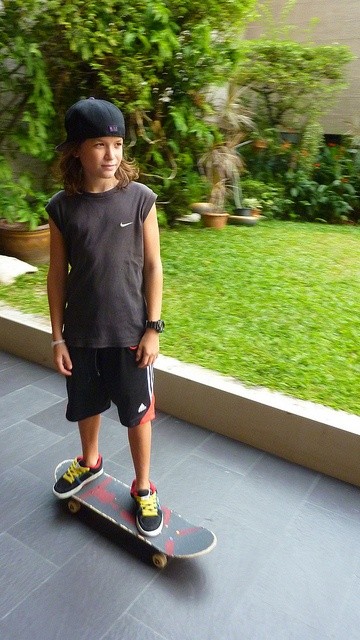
143, 318, 167, 334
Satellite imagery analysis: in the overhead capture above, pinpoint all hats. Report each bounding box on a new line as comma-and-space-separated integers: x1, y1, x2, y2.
56, 97, 125, 150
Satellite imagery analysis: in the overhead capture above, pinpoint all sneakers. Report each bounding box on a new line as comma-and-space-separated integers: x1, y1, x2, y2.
53, 455, 104, 498
130, 478, 164, 537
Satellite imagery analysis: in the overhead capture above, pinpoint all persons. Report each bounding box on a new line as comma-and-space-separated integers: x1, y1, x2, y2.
46, 98, 164, 538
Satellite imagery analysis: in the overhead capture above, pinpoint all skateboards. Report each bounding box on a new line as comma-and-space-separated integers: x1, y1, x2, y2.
54, 459, 217, 568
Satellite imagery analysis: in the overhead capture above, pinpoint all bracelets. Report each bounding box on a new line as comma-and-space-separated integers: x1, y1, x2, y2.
50, 339, 66, 347
